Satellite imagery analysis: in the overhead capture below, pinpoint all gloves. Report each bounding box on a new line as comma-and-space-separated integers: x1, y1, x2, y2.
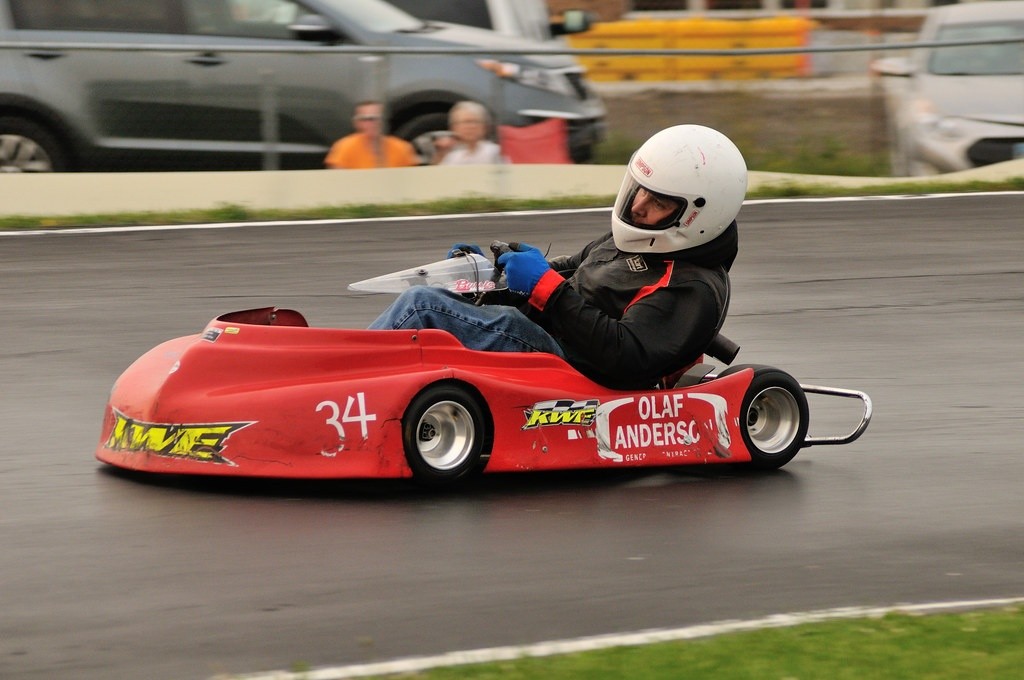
447, 242, 486, 262
498, 241, 550, 297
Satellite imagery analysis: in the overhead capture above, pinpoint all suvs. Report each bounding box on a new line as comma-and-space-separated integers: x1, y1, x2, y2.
1, 0, 608, 174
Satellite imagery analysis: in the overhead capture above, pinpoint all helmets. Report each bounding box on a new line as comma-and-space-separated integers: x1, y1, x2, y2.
611, 124, 748, 253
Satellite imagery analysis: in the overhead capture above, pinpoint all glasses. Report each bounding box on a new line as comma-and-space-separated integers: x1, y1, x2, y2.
353, 115, 381, 122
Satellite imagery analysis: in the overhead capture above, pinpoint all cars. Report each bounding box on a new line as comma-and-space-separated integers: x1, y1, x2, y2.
868, 1, 1024, 176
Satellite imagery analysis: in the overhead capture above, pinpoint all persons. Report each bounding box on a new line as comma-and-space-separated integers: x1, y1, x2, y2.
364, 125, 749, 389
324, 96, 423, 169
428, 100, 513, 165
